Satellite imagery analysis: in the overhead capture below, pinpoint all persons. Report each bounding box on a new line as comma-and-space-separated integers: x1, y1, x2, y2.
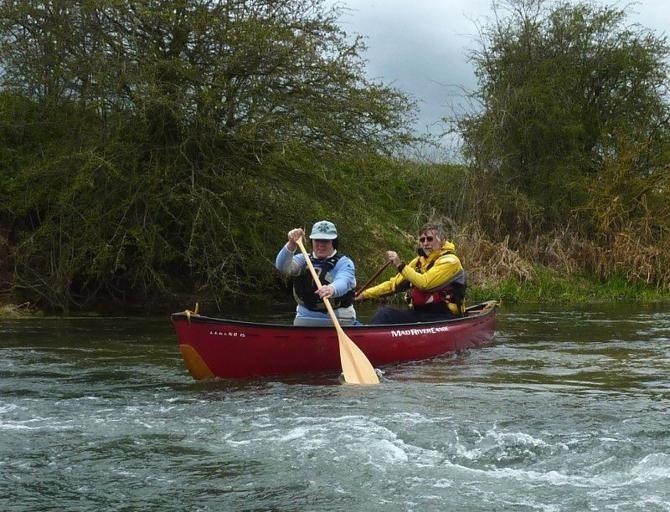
352, 222, 470, 324
274, 219, 365, 326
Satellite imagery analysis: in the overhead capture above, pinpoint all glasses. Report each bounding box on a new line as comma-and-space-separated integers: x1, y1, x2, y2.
420, 236, 433, 243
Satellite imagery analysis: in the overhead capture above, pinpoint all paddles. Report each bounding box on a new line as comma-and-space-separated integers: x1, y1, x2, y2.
296, 235, 380, 384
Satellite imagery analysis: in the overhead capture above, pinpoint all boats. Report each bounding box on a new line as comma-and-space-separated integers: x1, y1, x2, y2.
170, 300, 497, 383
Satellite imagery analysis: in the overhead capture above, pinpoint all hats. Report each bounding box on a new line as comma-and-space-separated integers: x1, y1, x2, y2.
309, 221, 338, 240
